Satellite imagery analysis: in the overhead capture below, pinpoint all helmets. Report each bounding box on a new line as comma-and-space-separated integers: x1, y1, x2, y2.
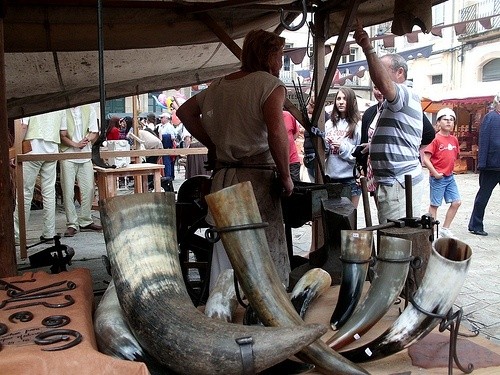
435, 108, 456, 122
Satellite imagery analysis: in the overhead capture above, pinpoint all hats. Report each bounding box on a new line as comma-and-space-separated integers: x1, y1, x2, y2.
158, 112, 170, 119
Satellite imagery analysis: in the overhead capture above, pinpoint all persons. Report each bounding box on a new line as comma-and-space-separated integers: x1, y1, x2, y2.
423, 107, 462, 242
105, 112, 212, 194
468, 94, 500, 235
351, 16, 436, 225
282, 102, 317, 182
5, 104, 103, 244
175, 29, 294, 296
324, 87, 363, 230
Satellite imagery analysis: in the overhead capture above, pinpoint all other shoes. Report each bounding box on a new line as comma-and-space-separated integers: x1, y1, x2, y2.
63, 227, 77, 237
439, 227, 459, 240
40, 236, 54, 244
468, 227, 489, 236
79, 222, 103, 232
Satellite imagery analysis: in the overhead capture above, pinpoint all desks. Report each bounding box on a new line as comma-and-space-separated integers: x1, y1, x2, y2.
93, 162, 165, 200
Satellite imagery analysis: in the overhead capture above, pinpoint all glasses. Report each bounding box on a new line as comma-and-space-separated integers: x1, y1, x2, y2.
160, 117, 164, 119
